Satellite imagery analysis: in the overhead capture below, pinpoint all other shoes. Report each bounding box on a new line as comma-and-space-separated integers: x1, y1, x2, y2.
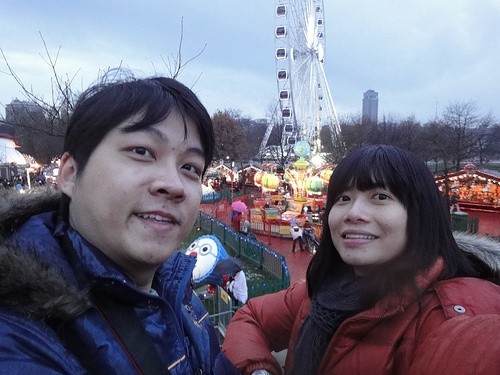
292, 250, 295, 252
302, 249, 304, 252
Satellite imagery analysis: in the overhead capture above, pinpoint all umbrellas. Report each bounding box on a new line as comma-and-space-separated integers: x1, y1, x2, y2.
231, 200, 247, 212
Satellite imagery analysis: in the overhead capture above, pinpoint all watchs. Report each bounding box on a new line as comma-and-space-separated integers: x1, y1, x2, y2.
252, 369, 270, 375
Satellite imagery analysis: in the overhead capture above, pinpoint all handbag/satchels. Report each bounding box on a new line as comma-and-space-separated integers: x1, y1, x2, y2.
294, 228, 299, 231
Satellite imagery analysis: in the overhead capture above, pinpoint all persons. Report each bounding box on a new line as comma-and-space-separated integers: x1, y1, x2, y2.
204, 174, 227, 191
438, 174, 498, 215
2, 169, 47, 190
221, 144, 500, 375
229, 208, 251, 236
290, 204, 327, 252
0, 77, 237, 375
